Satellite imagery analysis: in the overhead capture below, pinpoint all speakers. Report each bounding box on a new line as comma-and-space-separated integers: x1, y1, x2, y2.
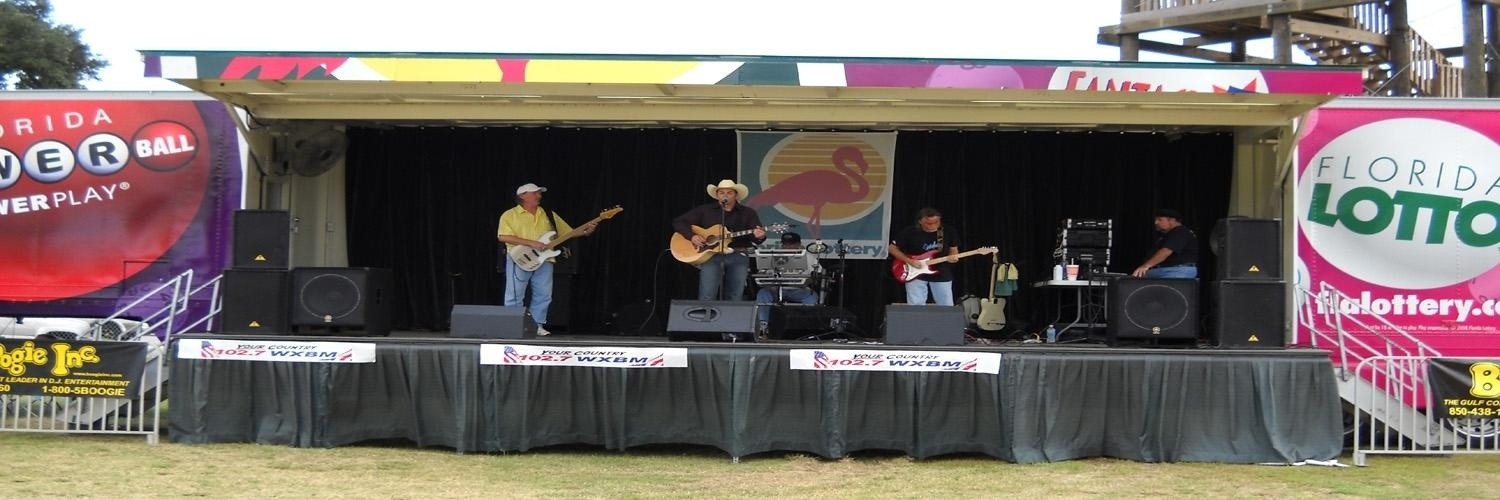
1215, 218, 1282, 281
220, 269, 291, 336
1104, 276, 1200, 348
881, 305, 965, 346
496, 239, 585, 276
666, 299, 761, 342
450, 304, 538, 339
232, 209, 290, 270
1207, 281, 1287, 350
288, 267, 394, 337
523, 274, 574, 329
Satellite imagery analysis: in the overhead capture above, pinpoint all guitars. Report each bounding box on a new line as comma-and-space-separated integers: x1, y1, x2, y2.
890, 245, 999, 284
510, 204, 624, 271
670, 221, 790, 264
977, 251, 1006, 331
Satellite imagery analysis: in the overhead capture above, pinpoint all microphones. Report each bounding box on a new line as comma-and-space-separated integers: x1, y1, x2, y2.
838, 239, 843, 244
720, 197, 729, 205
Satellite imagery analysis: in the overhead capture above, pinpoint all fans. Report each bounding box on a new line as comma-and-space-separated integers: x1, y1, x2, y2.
268, 130, 344, 177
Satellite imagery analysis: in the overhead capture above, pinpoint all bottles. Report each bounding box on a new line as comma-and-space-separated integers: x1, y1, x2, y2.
1053, 264, 1064, 281
1046, 324, 1056, 344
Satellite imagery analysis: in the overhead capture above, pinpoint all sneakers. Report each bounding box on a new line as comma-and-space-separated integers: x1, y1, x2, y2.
537, 326, 551, 336
759, 328, 770, 339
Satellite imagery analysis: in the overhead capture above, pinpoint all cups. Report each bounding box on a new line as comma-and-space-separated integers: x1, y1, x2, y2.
1065, 264, 1080, 281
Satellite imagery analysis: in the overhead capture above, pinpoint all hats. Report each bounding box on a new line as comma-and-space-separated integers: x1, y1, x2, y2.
1156, 207, 1181, 218
780, 232, 801, 243
516, 183, 548, 195
706, 179, 749, 200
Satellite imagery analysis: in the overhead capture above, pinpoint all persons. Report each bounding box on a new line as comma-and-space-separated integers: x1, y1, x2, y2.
497, 183, 598, 337
755, 232, 823, 336
672, 179, 767, 301
888, 207, 959, 306
1130, 208, 1199, 279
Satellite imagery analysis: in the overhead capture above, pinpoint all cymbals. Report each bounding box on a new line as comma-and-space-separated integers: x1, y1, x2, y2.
805, 242, 829, 254
